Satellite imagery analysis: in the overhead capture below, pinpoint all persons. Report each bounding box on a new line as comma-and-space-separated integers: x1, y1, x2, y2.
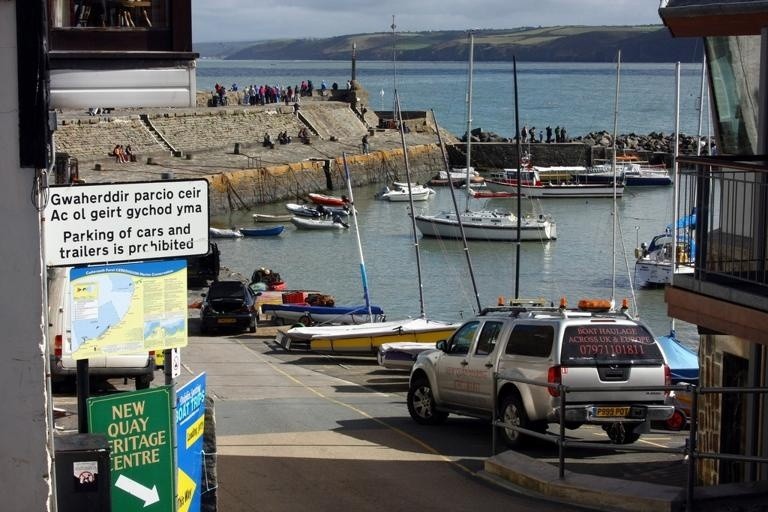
263, 128, 311, 147
215, 83, 225, 96
89, 108, 115, 116
361, 104, 367, 121
299, 79, 315, 97
403, 122, 406, 132
320, 80, 327, 96
332, 81, 338, 90
521, 125, 566, 143
243, 83, 299, 104
113, 144, 137, 163
346, 80, 352, 90
232, 82, 238, 92
362, 134, 369, 154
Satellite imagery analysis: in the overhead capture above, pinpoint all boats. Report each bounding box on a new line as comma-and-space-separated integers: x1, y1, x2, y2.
250, 267, 467, 371
375, 181, 435, 201
424, 163, 485, 188
409, 204, 555, 242
209, 191, 358, 236
633, 207, 697, 284
488, 156, 676, 198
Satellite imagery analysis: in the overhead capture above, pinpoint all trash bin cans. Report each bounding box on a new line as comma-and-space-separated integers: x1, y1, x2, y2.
213, 96, 220, 107
55, 152, 78, 185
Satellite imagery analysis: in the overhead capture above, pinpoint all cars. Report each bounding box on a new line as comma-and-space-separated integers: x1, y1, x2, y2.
160, 242, 221, 288
406, 300, 678, 447
200, 278, 261, 332
